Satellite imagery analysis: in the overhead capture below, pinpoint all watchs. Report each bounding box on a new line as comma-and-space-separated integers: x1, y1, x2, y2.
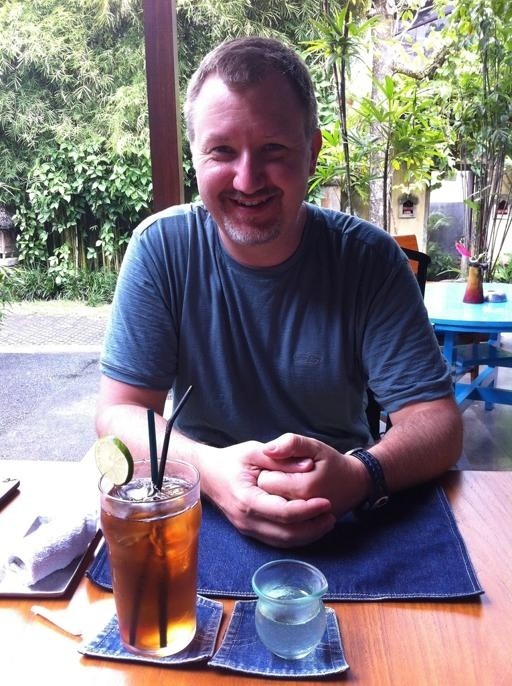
343, 444, 393, 519
93, 37, 464, 550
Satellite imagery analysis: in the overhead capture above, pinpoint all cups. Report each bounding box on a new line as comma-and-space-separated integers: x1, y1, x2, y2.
251, 560, 329, 658
95, 458, 197, 656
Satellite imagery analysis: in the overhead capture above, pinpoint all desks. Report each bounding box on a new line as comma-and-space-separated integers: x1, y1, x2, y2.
0, 455, 511, 686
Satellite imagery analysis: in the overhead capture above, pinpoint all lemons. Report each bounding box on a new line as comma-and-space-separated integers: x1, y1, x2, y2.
92, 436, 136, 488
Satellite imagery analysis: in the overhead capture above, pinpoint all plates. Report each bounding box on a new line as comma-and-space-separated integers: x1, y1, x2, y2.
1, 514, 100, 598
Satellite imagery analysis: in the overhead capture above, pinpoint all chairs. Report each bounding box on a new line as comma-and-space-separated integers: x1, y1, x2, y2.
364, 238, 432, 435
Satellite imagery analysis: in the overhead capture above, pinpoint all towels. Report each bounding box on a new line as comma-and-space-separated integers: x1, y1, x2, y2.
10, 513, 94, 586
